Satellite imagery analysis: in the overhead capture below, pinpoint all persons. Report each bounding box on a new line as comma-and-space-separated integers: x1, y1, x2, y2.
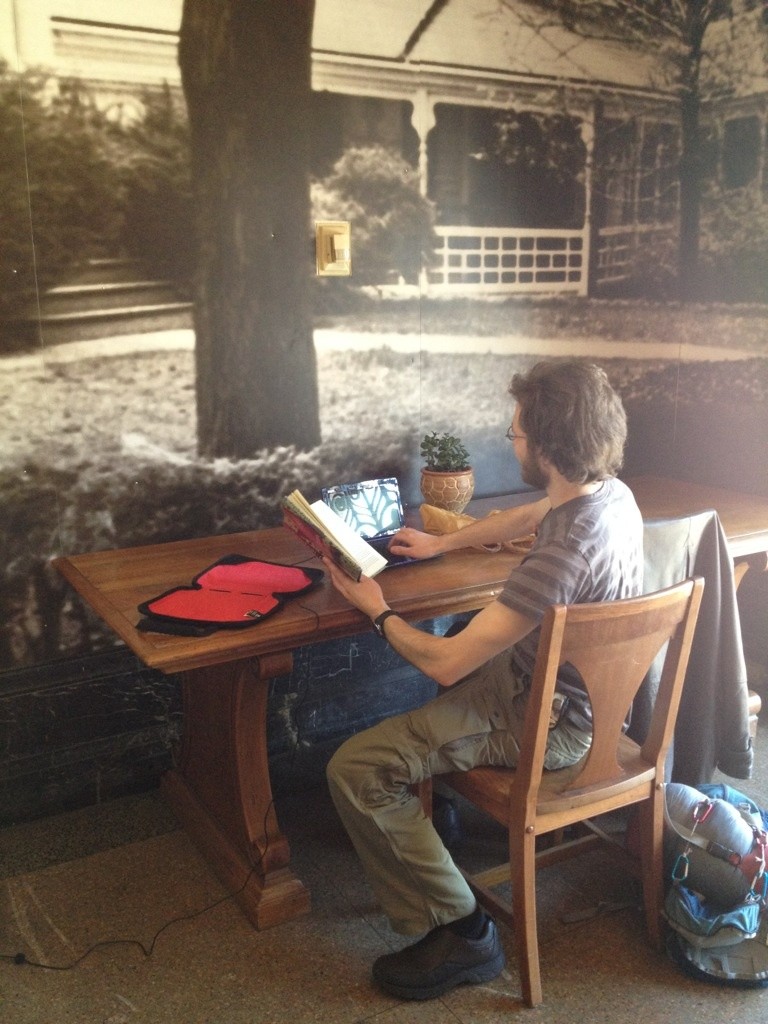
323, 361, 644, 1001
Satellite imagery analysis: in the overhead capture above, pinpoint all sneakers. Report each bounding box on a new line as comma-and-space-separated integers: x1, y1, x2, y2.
429, 792, 460, 847
370, 911, 504, 1000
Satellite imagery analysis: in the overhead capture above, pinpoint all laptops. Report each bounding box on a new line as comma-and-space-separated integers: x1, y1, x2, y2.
322, 476, 443, 570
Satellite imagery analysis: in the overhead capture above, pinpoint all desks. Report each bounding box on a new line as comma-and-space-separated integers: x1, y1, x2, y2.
53, 471, 768, 933
419, 430, 475, 516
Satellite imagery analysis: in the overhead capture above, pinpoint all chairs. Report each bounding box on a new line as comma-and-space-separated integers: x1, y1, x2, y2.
405, 576, 705, 1009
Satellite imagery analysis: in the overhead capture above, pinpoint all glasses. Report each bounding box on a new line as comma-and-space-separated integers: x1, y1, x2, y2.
505, 425, 528, 442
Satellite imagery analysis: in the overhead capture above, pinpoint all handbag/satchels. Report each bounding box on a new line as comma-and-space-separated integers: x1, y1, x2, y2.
662, 775, 768, 987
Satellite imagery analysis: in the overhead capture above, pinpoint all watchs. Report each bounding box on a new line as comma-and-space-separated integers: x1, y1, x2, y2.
372, 609, 402, 641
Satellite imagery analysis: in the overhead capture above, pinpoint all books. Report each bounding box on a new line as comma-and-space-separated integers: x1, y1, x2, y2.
279, 487, 388, 583
